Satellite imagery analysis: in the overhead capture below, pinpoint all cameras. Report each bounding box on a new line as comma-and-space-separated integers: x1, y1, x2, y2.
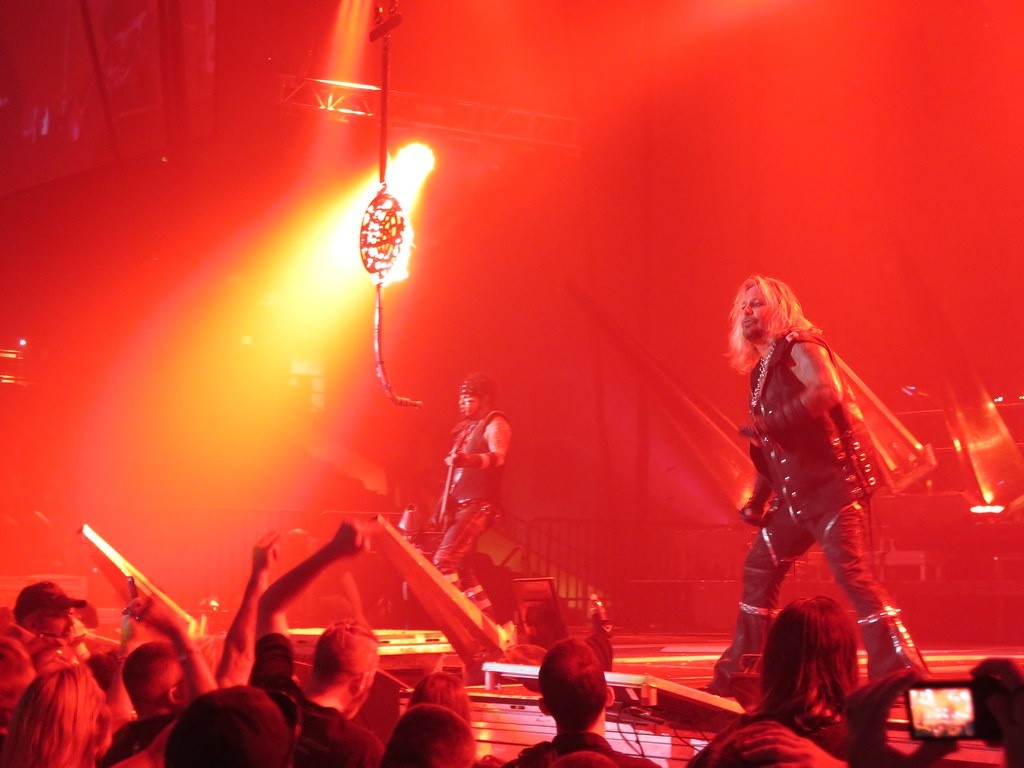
905, 682, 1002, 741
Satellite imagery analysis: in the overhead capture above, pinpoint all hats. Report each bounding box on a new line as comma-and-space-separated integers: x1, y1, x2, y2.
457, 371, 496, 398
14, 581, 88, 626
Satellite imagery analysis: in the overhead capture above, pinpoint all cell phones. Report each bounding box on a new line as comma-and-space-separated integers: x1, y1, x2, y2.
126, 576, 142, 622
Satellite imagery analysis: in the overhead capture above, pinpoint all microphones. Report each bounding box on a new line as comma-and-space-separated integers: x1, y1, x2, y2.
394, 397, 423, 407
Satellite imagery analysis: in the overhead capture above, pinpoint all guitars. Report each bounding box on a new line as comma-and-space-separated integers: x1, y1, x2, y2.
424, 429, 467, 539
828, 401, 878, 499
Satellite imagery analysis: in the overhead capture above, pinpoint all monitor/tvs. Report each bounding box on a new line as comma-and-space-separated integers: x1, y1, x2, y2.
513, 576, 568, 652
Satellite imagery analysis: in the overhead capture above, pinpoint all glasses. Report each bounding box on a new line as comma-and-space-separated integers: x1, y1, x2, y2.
31, 610, 75, 622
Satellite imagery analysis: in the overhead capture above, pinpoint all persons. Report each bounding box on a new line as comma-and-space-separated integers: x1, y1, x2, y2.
706, 719, 851, 767
433, 371, 511, 618
841, 658, 1024, 768
14, 579, 87, 681
714, 274, 932, 683
166, 683, 295, 768
380, 703, 475, 767
545, 751, 621, 767
400, 672, 503, 767
505, 636, 662, 767
251, 518, 386, 767
99, 540, 277, 767
2, 595, 216, 768
688, 595, 865, 767
0, 634, 36, 766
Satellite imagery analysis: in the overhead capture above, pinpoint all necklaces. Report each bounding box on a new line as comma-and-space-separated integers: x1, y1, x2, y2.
749, 341, 777, 408
